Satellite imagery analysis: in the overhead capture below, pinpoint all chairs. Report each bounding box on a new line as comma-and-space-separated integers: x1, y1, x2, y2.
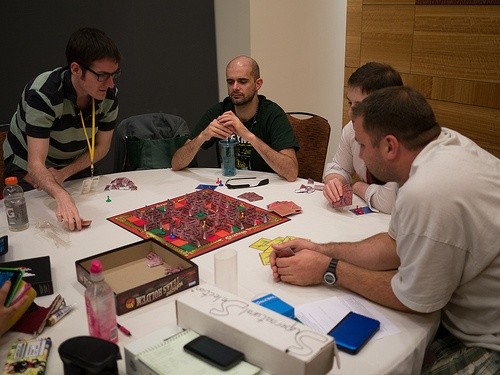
116, 113, 198, 171
284, 111, 330, 183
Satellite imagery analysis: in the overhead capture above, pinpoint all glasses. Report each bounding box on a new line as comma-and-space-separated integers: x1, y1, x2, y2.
79, 65, 120, 81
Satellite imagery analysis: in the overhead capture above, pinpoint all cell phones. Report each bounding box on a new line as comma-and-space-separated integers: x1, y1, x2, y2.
0, 235, 8, 256
184, 336, 245, 371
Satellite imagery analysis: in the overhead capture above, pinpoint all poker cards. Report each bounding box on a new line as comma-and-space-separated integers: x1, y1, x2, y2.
331, 183, 353, 208
267, 200, 302, 217
248, 236, 295, 266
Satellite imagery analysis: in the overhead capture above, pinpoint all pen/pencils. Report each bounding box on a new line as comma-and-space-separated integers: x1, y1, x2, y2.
116, 322, 132, 337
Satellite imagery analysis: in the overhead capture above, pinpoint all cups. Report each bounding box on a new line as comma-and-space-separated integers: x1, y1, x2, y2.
219, 136, 239, 177
212, 248, 238, 297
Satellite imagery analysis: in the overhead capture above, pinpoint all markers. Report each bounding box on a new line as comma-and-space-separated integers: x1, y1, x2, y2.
47, 305, 73, 327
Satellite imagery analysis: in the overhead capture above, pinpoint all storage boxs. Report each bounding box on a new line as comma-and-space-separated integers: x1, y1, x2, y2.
123, 323, 262, 375
74, 236, 200, 317
173, 282, 342, 375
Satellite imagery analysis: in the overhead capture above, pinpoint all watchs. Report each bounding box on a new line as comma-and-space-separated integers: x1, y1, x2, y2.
323, 257, 338, 286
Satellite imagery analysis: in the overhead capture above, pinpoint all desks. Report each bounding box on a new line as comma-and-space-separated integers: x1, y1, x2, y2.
0, 168, 441, 375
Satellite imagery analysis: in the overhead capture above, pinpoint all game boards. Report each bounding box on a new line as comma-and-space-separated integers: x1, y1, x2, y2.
106, 188, 292, 259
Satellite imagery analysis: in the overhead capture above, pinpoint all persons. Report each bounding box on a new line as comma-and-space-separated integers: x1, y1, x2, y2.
323, 62, 406, 215
269, 84, 500, 375
1, 27, 122, 231
171, 56, 300, 183
1, 280, 30, 333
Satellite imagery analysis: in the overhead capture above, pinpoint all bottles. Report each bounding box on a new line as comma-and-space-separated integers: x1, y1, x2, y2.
83, 260, 119, 345
3, 176, 30, 231
